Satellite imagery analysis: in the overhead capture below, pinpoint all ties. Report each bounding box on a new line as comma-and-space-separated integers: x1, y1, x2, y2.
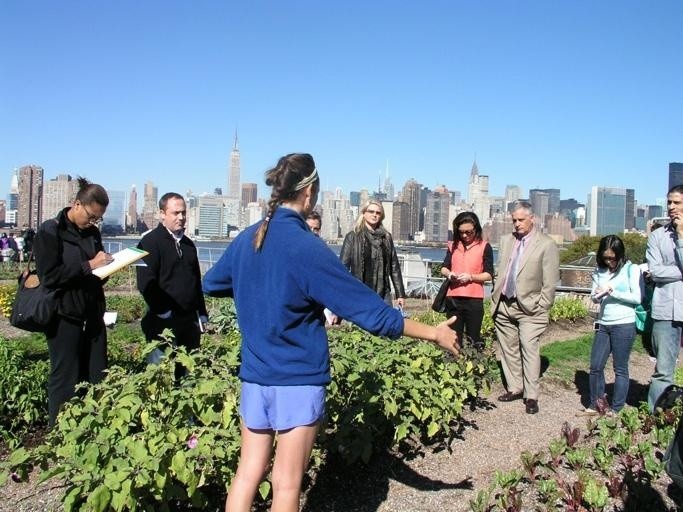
505, 237, 525, 300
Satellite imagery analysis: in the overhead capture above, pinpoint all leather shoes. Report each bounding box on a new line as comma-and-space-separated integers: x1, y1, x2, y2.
524, 398, 538, 413
498, 392, 523, 402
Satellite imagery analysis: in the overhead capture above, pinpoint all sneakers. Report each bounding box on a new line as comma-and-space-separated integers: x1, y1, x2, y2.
585, 407, 599, 413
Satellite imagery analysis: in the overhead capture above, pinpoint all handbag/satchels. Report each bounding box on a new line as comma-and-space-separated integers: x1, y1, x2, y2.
635, 286, 652, 335
9, 269, 62, 333
432, 280, 451, 312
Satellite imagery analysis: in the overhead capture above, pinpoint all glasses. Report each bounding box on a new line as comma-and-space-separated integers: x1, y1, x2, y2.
458, 226, 476, 236
79, 200, 103, 224
364, 209, 381, 215
601, 255, 617, 262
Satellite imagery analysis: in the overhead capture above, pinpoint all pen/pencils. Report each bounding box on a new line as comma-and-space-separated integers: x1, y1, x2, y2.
94, 239, 106, 253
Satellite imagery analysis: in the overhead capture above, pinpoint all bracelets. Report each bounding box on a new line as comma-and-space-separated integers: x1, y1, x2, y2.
468, 273, 472, 282
447, 270, 453, 281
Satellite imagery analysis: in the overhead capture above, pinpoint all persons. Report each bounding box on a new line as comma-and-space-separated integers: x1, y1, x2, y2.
588, 234, 641, 432
31, 171, 119, 449
490, 198, 557, 417
0, 227, 39, 262
198, 151, 464, 512
644, 184, 683, 411
327, 198, 406, 326
304, 213, 326, 324
132, 192, 213, 388
438, 210, 493, 352
